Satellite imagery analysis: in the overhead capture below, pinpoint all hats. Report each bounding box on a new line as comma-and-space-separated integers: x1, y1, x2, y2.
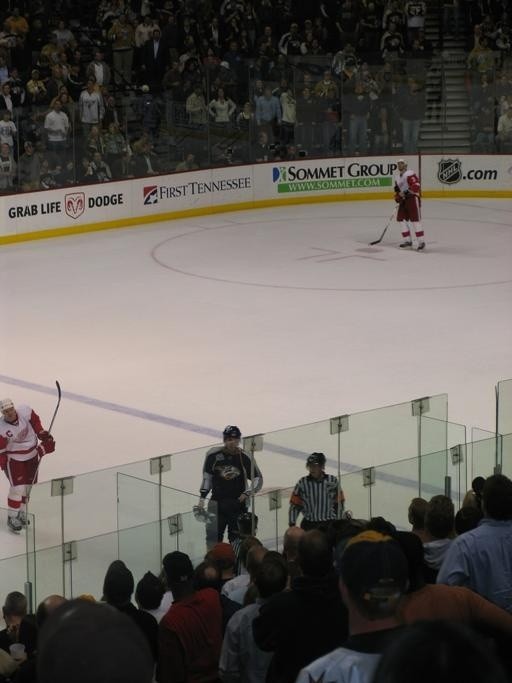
213, 543, 235, 559
337, 530, 407, 601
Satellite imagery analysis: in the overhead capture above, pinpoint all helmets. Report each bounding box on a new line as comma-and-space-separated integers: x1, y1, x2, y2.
307, 453, 325, 465
224, 426, 241, 438
0, 400, 14, 413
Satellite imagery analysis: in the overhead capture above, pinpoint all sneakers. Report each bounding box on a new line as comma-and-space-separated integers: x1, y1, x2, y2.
8, 514, 30, 530
400, 241, 424, 249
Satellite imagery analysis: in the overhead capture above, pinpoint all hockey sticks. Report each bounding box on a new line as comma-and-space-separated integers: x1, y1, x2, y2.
29, 380, 62, 496
369, 204, 400, 245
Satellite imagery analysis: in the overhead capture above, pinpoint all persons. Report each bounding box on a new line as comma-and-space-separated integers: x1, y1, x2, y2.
394, 159, 425, 250
444, 1, 512, 154
1, 399, 55, 530
198, 474, 510, 682
0, 551, 223, 683
1, 1, 429, 193
198, 426, 263, 552
288, 453, 345, 531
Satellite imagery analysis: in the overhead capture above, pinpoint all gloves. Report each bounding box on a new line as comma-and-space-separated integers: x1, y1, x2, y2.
36, 430, 55, 457
